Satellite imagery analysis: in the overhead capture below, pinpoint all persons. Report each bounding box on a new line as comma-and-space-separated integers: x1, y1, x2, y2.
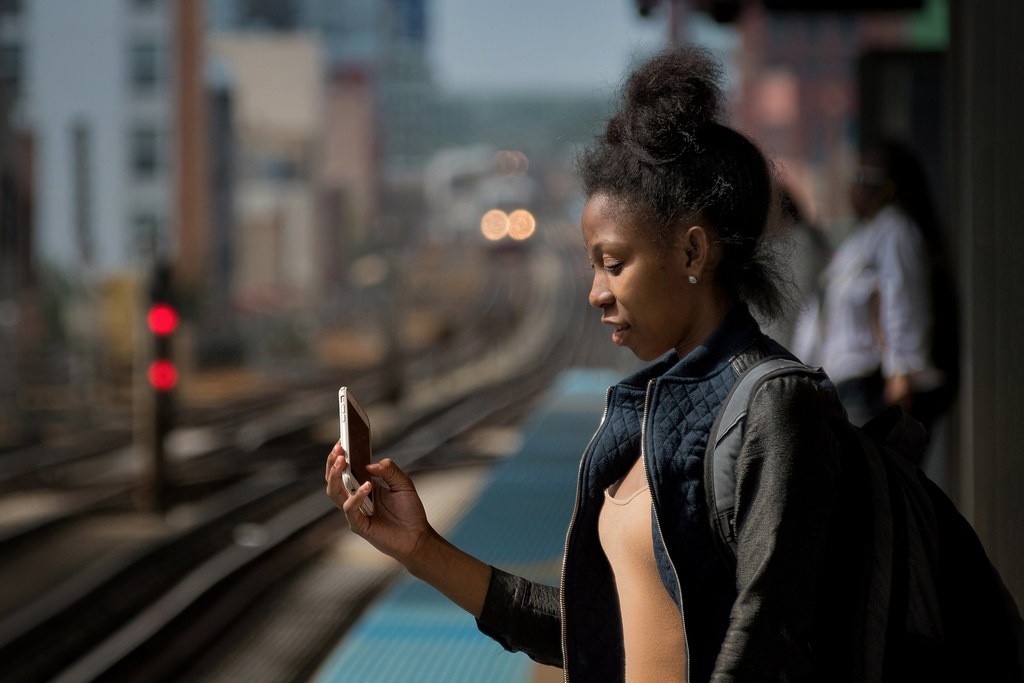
816, 152, 933, 415
750, 187, 833, 354
322, 44, 879, 683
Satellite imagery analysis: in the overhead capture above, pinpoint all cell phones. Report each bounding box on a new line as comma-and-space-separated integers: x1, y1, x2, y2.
338, 386, 376, 517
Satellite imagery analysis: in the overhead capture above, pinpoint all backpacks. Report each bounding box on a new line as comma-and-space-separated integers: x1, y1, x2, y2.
703, 334, 1024, 683
